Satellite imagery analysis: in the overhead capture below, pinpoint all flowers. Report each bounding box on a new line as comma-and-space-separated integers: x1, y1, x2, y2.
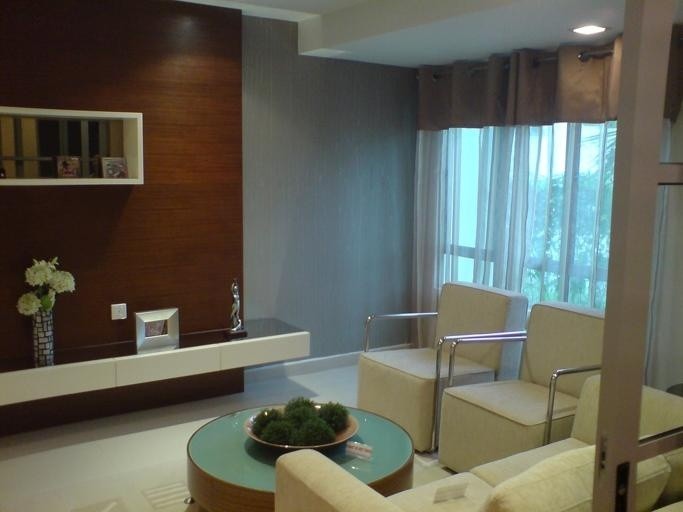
17, 256, 76, 317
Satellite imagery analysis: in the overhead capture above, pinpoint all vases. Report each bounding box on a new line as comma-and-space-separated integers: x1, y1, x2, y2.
33, 310, 53, 359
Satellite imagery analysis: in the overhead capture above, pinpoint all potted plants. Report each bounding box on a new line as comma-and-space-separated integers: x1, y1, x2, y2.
244, 397, 359, 449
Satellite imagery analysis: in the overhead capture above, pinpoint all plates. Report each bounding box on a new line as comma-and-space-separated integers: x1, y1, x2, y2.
245, 405, 358, 449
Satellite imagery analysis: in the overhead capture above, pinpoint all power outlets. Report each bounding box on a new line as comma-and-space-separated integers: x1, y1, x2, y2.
111, 303, 127, 321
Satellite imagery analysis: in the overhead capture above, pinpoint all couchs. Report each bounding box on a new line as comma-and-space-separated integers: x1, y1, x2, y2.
437, 301, 605, 474
274, 375, 683, 512
358, 282, 528, 451
